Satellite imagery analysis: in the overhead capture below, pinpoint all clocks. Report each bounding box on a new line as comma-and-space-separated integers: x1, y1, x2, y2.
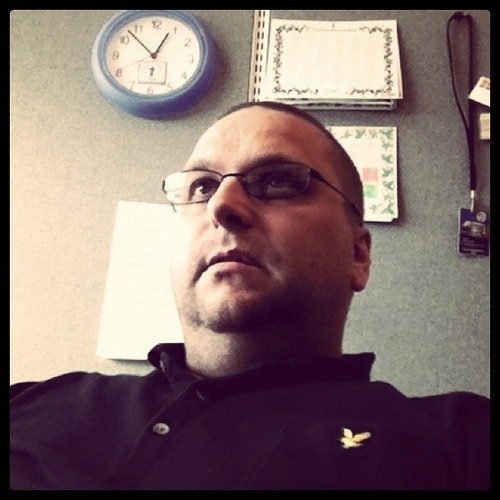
91, 10, 216, 119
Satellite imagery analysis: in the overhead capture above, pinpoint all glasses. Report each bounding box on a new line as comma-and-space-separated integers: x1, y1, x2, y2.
162, 160, 364, 225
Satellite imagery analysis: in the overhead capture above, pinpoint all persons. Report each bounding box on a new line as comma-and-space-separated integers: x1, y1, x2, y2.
10, 103, 491, 491
